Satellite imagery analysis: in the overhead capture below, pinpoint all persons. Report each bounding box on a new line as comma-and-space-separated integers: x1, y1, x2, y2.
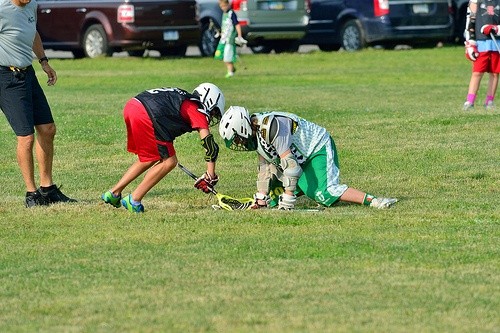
0, 0, 79, 209
463, 0, 500, 110
99, 83, 226, 213
219, 105, 401, 210
213, 0, 248, 79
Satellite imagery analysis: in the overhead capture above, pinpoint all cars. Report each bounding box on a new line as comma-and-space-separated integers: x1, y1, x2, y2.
443, 0, 471, 46
196, 0, 310, 57
36, 0, 201, 58
248, 0, 456, 51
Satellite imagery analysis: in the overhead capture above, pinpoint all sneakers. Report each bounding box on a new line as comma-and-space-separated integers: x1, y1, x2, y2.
119, 194, 144, 213
24, 184, 77, 208
101, 190, 122, 208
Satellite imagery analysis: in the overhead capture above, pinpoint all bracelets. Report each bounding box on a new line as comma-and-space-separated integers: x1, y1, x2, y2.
39, 55, 48, 63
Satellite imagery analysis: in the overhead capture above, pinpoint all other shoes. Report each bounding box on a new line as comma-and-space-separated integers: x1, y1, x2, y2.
225, 67, 237, 79
462, 102, 495, 111
370, 196, 398, 210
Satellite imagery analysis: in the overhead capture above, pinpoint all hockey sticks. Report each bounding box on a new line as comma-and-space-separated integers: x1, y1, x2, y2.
176, 163, 254, 212
208, 18, 248, 70
211, 205, 320, 212
490, 32, 500, 54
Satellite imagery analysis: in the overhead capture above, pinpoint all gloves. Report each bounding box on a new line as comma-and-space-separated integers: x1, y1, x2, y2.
250, 193, 270, 210
193, 171, 219, 194
480, 24, 498, 36
464, 40, 480, 62
277, 192, 296, 211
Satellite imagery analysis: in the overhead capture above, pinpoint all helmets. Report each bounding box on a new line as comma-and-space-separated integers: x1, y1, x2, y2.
218, 105, 254, 152
190, 82, 226, 120
